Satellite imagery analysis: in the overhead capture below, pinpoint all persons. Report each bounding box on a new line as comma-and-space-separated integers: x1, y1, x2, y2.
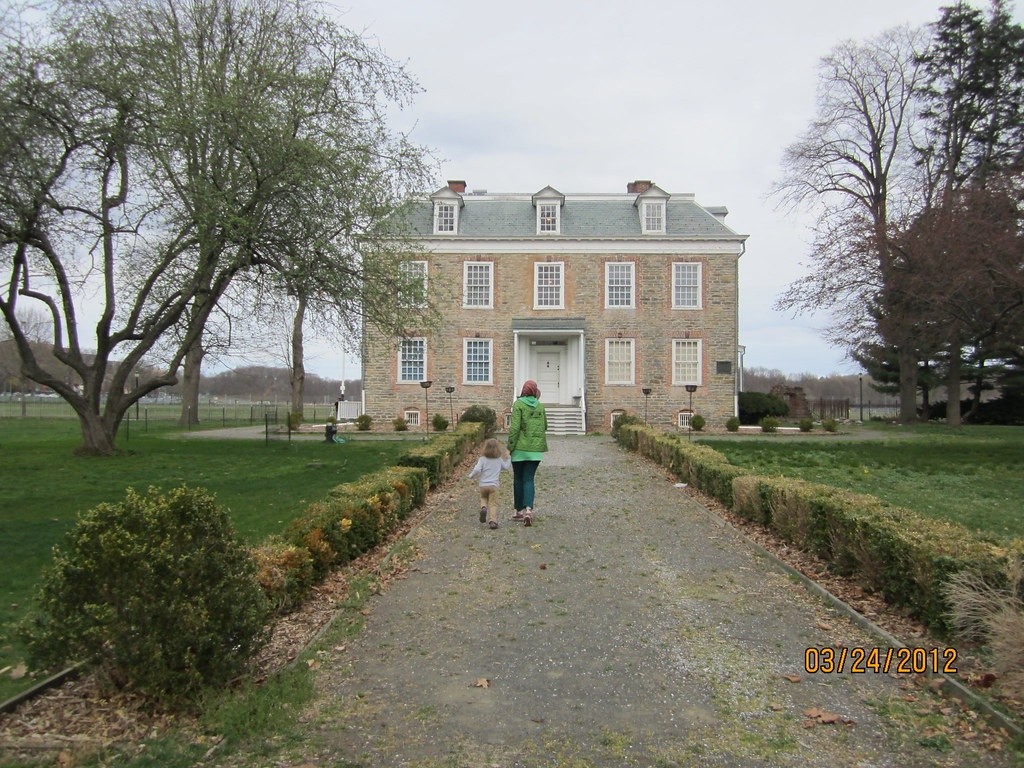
469, 438, 511, 528
504, 380, 549, 526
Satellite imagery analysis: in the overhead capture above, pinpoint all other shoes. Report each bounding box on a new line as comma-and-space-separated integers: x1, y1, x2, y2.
489, 520, 497, 529
479, 508, 487, 523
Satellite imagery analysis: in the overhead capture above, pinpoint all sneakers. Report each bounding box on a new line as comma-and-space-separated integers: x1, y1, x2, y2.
524, 512, 532, 526
508, 511, 524, 521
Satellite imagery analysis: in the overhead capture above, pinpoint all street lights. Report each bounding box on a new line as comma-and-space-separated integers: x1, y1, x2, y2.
858, 373, 865, 420
134, 369, 141, 421
